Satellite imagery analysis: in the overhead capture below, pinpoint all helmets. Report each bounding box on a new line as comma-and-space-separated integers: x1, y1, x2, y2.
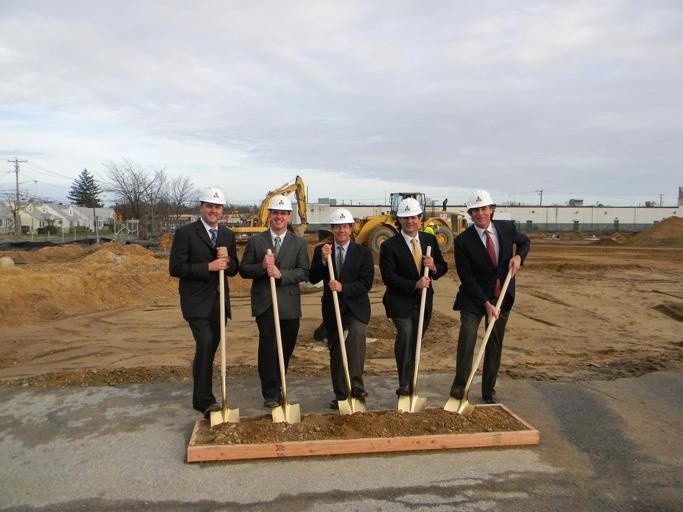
465, 189, 493, 212
198, 186, 227, 206
331, 207, 355, 226
268, 194, 293, 211
397, 197, 422, 219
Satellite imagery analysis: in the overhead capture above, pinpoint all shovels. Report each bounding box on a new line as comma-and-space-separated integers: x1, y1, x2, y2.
397, 245, 431, 415
444, 264, 514, 417
211, 269, 239, 428
327, 254, 368, 416
266, 248, 300, 424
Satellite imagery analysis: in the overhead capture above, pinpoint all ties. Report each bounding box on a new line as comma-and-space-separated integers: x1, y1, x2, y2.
207, 227, 219, 247
409, 238, 425, 277
271, 237, 280, 257
483, 229, 503, 298
332, 245, 344, 276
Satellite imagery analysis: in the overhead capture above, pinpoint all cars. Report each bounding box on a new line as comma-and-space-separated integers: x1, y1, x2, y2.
125, 230, 161, 250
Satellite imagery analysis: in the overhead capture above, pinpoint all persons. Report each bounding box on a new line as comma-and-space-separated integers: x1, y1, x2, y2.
380, 198, 448, 397
309, 205, 375, 409
450, 189, 531, 403
169, 187, 239, 418
239, 196, 310, 406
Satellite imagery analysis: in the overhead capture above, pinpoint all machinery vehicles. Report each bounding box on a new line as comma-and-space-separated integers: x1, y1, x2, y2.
352, 193, 467, 256
224, 175, 308, 244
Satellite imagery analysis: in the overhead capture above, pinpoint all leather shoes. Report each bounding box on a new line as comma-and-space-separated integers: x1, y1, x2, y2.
481, 388, 497, 405
330, 396, 345, 407
395, 382, 412, 396
262, 393, 280, 409
195, 397, 222, 418
448, 382, 469, 400
351, 384, 367, 398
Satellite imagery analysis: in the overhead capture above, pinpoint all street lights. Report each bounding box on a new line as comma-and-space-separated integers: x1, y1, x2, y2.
15, 158, 39, 242
